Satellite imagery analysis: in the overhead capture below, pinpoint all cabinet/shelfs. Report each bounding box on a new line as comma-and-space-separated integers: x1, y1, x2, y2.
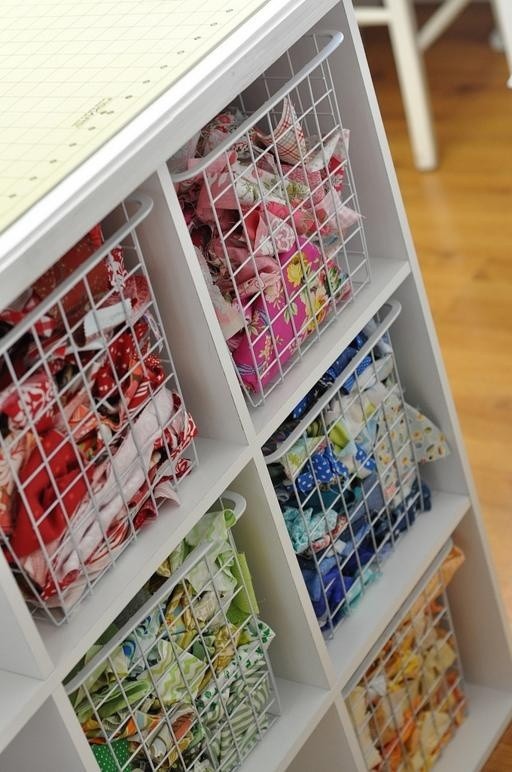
0, 0, 512, 771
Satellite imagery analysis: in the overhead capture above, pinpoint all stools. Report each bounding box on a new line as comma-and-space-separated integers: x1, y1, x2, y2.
352, 0, 512, 172
